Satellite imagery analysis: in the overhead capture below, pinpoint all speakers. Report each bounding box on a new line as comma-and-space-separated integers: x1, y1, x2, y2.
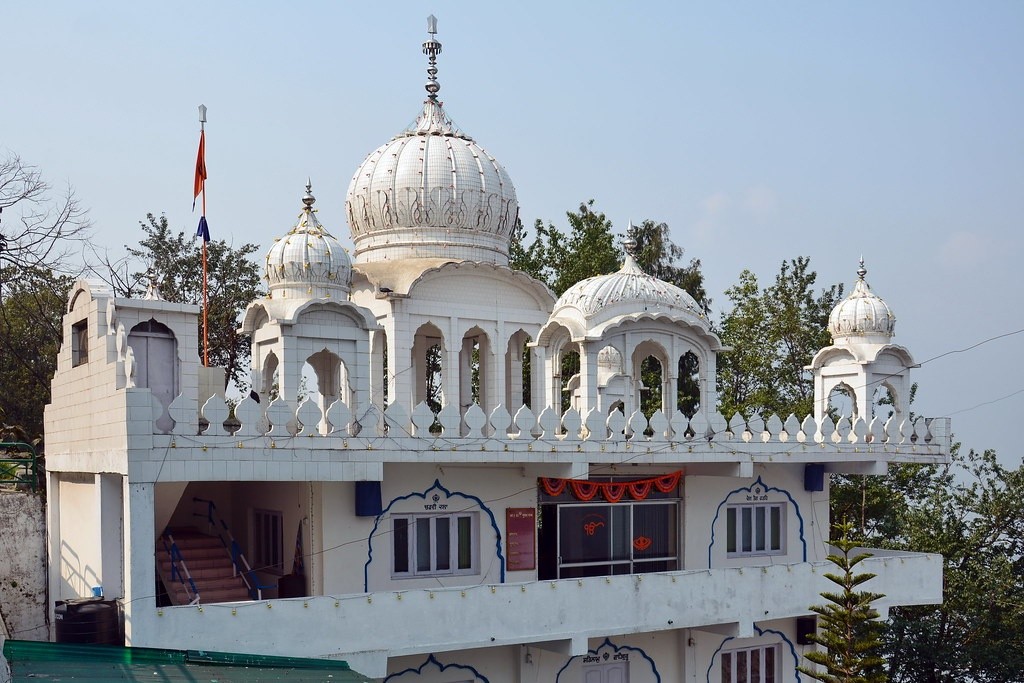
804, 464, 824, 491
355, 481, 382, 517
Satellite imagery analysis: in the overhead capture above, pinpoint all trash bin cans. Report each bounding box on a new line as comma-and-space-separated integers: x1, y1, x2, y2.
279, 572, 306, 599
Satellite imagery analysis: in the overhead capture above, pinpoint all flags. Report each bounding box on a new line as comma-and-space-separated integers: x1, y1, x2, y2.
192, 130, 207, 212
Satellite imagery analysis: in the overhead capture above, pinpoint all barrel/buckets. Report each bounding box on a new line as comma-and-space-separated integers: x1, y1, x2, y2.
92, 587, 101, 597
